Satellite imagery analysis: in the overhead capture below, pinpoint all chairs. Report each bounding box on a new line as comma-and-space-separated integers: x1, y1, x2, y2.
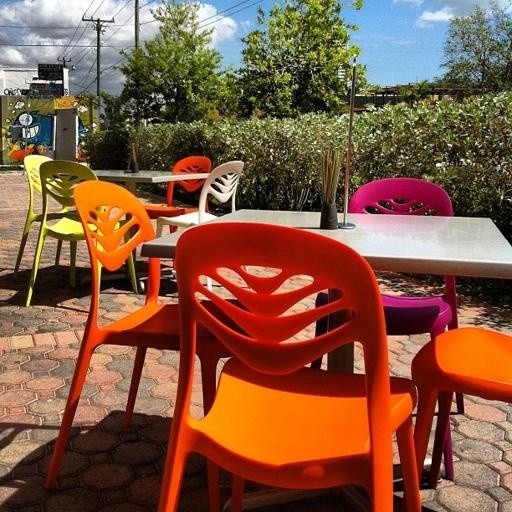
157, 221, 422, 510
26, 160, 139, 307
312, 177, 466, 482
141, 155, 212, 269
154, 160, 245, 292
13, 154, 83, 287
45, 180, 257, 510
410, 327, 511, 490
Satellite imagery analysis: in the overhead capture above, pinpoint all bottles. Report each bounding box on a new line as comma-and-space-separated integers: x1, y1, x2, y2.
127, 143, 139, 173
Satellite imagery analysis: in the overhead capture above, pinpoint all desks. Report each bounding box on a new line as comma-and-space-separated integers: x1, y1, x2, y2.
140, 208, 510, 374
57, 170, 211, 272
